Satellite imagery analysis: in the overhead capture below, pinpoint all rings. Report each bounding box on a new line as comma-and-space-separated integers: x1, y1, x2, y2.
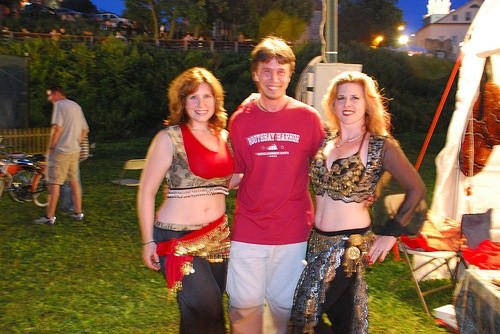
376, 249, 382, 253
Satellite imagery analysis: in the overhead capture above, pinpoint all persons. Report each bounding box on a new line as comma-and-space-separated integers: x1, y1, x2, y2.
50, 28, 68, 40
183, 33, 194, 41
136, 68, 239, 334
2, 24, 11, 41
33, 86, 91, 224
197, 35, 207, 47
239, 69, 425, 334
21, 27, 31, 38
165, 35, 376, 334
59, 134, 89, 214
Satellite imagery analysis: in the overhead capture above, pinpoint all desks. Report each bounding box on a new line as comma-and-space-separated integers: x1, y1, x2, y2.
451, 267, 500, 334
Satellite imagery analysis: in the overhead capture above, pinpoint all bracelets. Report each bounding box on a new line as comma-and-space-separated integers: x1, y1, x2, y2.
142, 240, 156, 245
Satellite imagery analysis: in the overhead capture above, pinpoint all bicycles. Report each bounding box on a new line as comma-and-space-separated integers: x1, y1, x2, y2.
0, 135, 61, 208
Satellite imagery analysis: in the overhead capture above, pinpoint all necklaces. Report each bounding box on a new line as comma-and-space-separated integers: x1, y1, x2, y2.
336, 134, 361, 148
258, 100, 286, 112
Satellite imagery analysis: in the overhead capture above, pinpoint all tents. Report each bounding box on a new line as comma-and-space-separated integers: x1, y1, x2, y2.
412, 0, 500, 285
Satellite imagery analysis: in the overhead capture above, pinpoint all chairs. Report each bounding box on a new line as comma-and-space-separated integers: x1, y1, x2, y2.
109, 158, 146, 210
384, 193, 460, 315
450, 207, 493, 289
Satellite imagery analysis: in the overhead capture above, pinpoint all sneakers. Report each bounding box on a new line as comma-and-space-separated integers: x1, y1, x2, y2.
71, 213, 84, 219
34, 215, 56, 224
